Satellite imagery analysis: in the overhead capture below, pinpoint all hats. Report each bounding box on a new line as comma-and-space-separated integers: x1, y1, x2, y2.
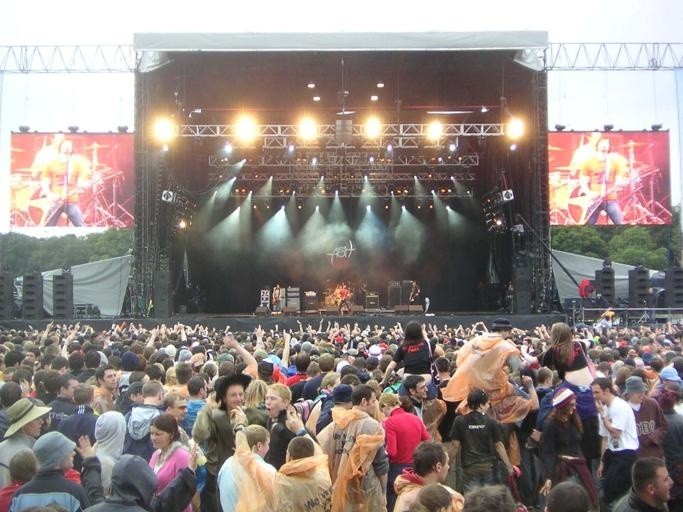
331, 384, 354, 404
3, 396, 53, 442
32, 431, 77, 473
552, 386, 576, 410
212, 372, 253, 403
0, 318, 683, 388
622, 375, 649, 396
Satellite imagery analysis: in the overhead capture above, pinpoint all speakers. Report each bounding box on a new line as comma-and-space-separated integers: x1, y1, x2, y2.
596, 270, 615, 308
0, 274, 14, 320
365, 296, 379, 308
255, 307, 341, 316
395, 304, 425, 315
664, 269, 683, 308
513, 267, 532, 314
301, 297, 318, 310
153, 271, 174, 318
629, 270, 650, 308
52, 275, 74, 320
22, 274, 44, 320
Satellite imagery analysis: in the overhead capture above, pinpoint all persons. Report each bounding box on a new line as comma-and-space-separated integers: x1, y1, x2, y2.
41, 139, 96, 226
31, 133, 90, 176
568, 131, 627, 176
578, 138, 626, 225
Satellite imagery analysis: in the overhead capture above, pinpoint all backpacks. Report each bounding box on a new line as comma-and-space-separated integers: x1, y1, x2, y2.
291, 393, 329, 425
